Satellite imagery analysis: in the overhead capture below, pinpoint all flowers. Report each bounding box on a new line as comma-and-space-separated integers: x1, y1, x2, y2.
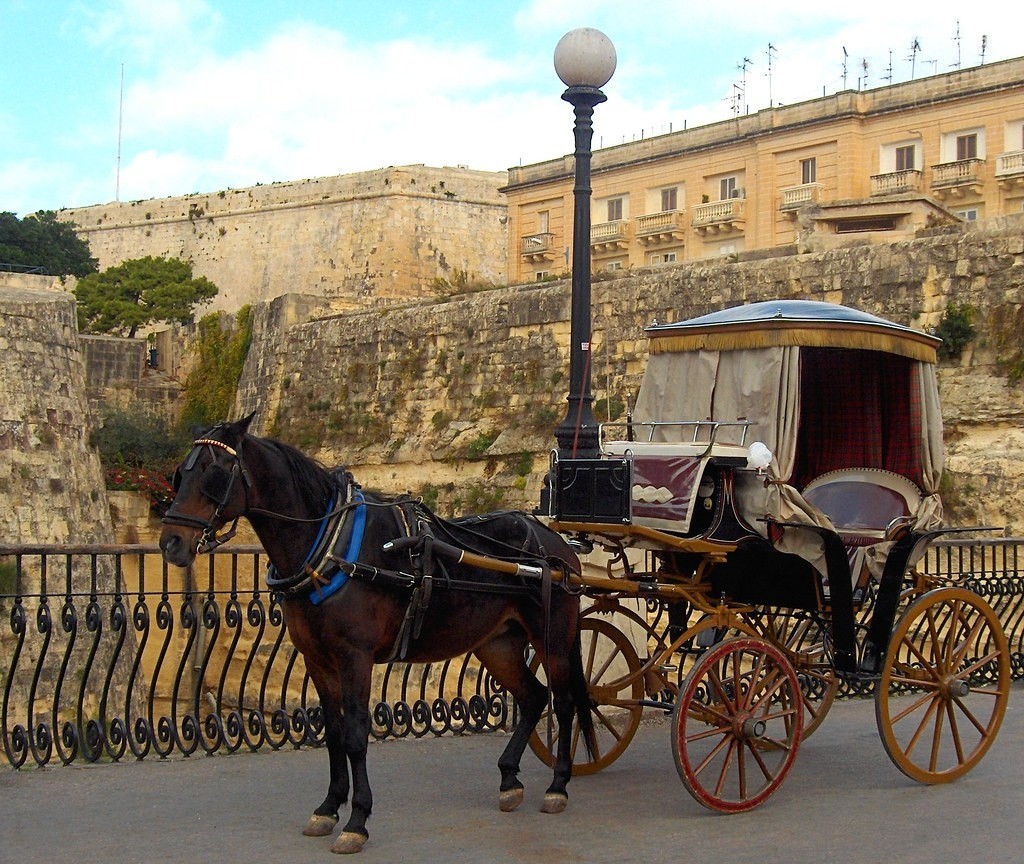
103, 463, 177, 506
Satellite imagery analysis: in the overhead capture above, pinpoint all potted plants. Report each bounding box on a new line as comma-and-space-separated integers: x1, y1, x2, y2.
702, 195, 710, 203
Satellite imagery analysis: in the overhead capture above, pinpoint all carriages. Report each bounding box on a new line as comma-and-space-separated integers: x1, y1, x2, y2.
158, 297, 1011, 854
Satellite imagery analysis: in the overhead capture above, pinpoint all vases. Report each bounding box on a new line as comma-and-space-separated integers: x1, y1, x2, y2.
106, 490, 151, 529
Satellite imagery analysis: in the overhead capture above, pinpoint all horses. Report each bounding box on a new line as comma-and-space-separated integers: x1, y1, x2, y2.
159, 410, 601, 854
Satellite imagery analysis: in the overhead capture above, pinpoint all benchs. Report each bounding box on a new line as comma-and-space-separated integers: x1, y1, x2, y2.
602, 416, 927, 610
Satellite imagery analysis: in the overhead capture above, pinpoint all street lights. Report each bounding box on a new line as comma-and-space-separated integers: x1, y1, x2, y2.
533, 27, 619, 518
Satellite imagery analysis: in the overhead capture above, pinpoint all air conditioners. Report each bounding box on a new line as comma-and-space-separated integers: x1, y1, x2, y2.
731, 188, 745, 199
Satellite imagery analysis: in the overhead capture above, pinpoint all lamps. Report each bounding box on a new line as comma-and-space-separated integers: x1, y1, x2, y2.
499, 215, 508, 224
